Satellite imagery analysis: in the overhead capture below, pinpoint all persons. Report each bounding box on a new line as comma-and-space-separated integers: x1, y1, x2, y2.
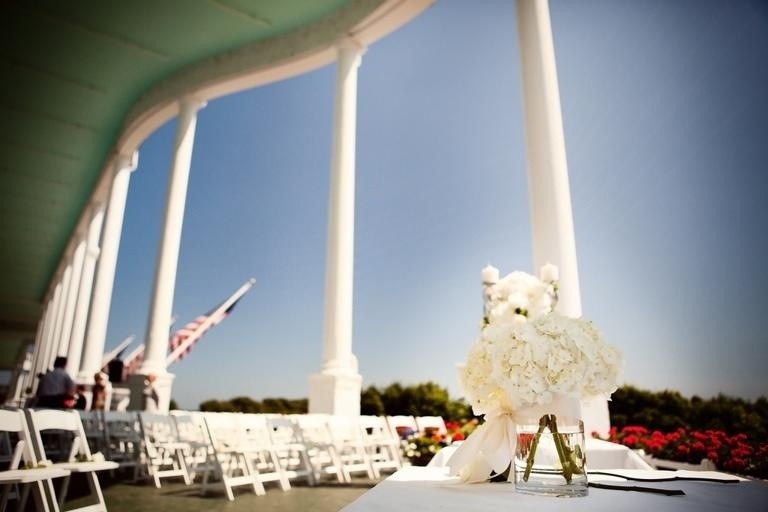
35, 355, 74, 410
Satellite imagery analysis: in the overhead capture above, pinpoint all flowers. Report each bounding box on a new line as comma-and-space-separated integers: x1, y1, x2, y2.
460, 316, 637, 483
478, 261, 562, 315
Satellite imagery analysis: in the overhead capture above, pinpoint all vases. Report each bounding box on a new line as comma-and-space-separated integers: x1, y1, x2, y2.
503, 401, 595, 498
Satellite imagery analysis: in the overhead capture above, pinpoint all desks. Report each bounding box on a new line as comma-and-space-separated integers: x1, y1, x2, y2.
342, 465, 768, 510
428, 436, 653, 469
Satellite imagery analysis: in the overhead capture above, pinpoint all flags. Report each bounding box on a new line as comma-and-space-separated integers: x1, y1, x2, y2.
74, 279, 253, 411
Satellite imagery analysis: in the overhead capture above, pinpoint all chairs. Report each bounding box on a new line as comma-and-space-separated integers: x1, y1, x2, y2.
1, 398, 451, 510
